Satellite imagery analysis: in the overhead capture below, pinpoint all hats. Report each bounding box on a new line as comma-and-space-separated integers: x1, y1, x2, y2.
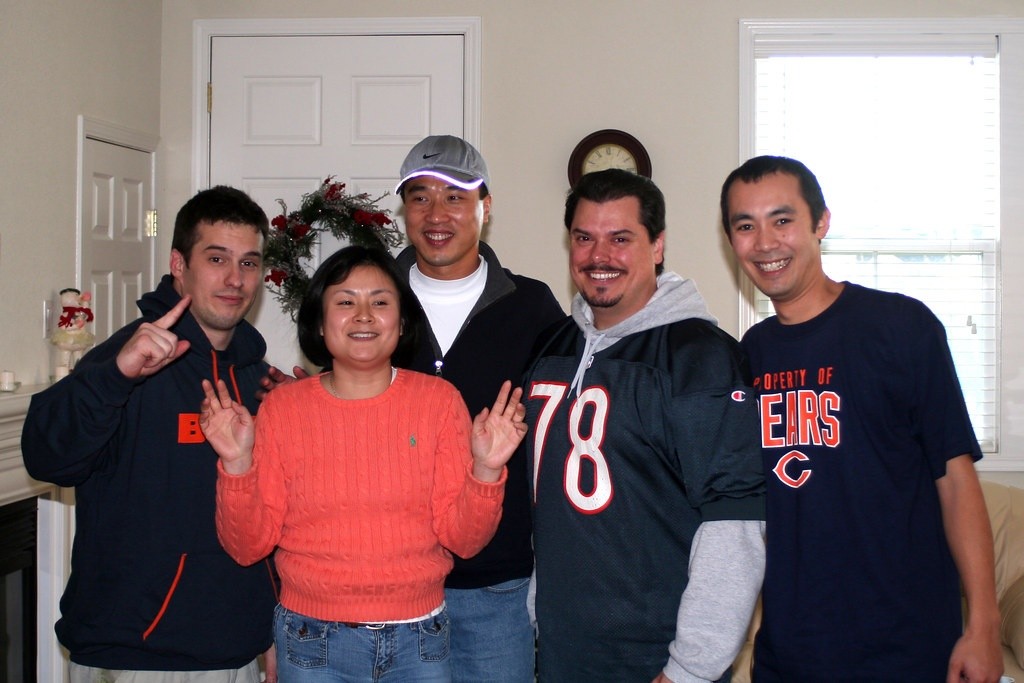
394, 134, 490, 198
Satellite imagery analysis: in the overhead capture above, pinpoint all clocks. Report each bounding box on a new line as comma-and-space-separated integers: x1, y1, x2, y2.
568, 129, 652, 188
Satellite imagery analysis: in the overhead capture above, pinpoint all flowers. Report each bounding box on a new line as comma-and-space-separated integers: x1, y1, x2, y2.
264, 173, 406, 330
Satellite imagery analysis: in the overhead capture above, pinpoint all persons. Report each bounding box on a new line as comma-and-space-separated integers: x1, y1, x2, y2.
522, 169, 771, 683
199, 245, 528, 683
256, 134, 567, 683
721, 155, 1006, 683
21, 186, 282, 683
